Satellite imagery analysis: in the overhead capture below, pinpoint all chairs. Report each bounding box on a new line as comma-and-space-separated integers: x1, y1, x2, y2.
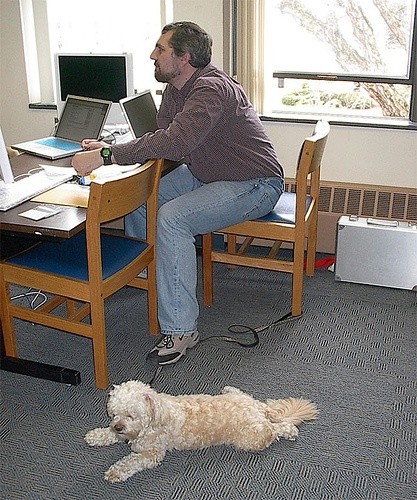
203, 118, 332, 318
0, 158, 164, 390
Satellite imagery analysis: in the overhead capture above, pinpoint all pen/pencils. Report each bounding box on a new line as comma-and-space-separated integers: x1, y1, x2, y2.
85, 137, 103, 151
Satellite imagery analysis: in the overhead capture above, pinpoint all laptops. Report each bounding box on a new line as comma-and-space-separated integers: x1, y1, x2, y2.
119, 89, 158, 140
12, 95, 113, 160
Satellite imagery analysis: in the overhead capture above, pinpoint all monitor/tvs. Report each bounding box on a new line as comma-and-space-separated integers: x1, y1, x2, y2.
53, 53, 133, 103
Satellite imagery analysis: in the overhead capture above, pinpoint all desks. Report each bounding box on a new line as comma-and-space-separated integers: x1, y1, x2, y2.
0, 148, 141, 386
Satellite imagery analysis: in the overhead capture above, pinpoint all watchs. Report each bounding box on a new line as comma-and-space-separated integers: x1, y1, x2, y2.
100, 147, 112, 165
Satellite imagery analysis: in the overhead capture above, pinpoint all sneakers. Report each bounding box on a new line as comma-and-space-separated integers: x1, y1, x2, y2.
146, 328, 200, 366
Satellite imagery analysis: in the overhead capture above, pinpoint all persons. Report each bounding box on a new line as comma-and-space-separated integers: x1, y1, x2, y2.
71, 21, 283, 365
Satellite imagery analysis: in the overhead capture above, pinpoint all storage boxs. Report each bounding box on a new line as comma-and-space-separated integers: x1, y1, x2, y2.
332, 214, 417, 293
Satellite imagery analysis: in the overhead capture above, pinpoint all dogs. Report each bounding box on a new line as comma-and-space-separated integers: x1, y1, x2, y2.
82, 379, 319, 484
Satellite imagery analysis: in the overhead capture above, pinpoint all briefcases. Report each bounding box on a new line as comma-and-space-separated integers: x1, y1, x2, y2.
334, 215, 417, 291
